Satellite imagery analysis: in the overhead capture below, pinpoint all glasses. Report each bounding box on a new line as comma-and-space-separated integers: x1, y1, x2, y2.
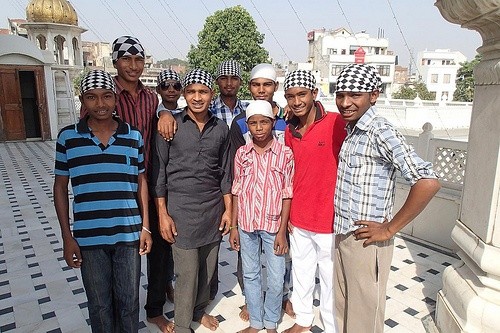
159, 82, 183, 91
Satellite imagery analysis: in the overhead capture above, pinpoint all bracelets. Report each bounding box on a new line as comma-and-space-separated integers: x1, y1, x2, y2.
142, 227, 152, 234
228, 225, 238, 230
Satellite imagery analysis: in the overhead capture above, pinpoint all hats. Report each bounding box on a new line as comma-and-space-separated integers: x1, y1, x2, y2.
215, 59, 243, 80
182, 68, 214, 91
245, 99, 274, 122
156, 68, 181, 85
282, 70, 316, 91
249, 63, 277, 83
80, 69, 117, 95
336, 63, 383, 93
111, 35, 145, 64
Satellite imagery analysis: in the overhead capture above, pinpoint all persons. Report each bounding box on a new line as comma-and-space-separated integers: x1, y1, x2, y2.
230, 63, 296, 322
154, 69, 183, 302
229, 100, 294, 333
54, 70, 153, 333
149, 69, 237, 333
281, 70, 348, 333
80, 35, 175, 333
333, 62, 441, 333
156, 60, 294, 142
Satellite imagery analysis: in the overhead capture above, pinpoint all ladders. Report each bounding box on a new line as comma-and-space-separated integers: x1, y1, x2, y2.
51, 67, 77, 130
